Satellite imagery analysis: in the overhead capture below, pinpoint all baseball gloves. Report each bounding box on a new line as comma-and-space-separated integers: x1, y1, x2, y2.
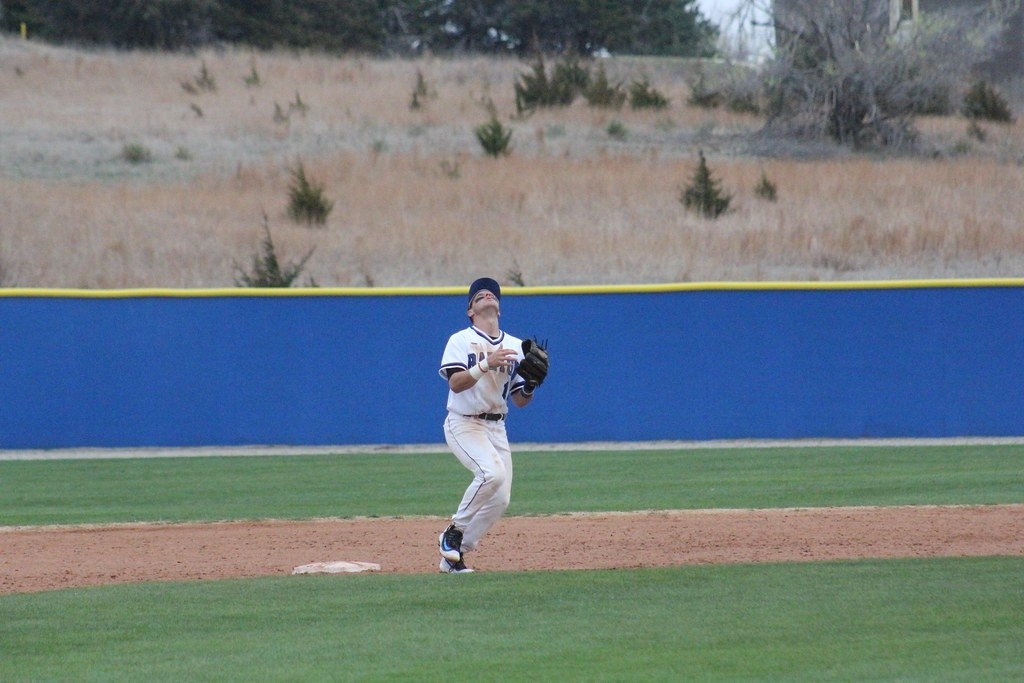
516, 338, 549, 387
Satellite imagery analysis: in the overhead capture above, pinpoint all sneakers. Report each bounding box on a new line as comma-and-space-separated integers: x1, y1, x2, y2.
438, 523, 475, 573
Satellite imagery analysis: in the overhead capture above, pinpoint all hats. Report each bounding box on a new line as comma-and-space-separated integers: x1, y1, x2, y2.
468, 278, 501, 323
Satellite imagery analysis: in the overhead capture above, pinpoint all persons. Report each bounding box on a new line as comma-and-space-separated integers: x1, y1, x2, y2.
438, 278, 536, 574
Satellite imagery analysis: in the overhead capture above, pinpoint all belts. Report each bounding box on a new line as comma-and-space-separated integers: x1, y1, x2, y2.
478, 413, 507, 421
